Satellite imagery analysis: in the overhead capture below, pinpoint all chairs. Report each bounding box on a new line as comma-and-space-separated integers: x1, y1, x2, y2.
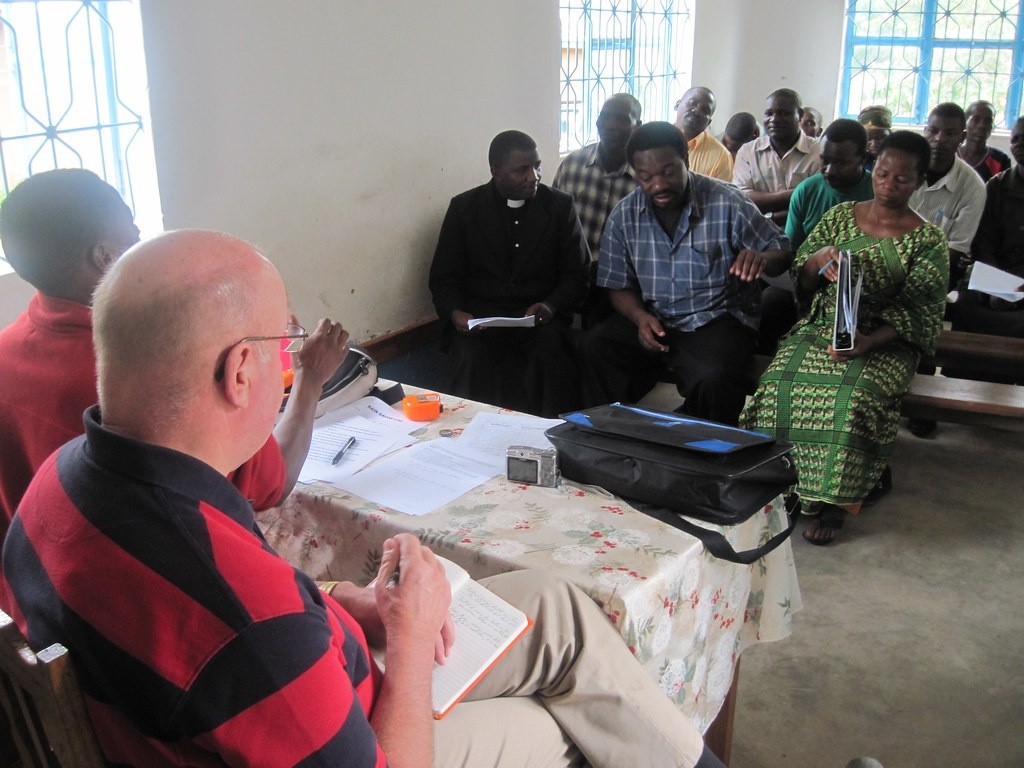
0, 608, 105, 768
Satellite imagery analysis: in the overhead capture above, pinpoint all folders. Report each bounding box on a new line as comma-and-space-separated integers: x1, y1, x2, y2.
833, 247, 854, 353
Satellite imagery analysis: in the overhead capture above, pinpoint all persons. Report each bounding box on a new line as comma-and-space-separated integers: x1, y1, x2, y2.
737, 131, 951, 545
0, 230, 882, 768
428, 128, 596, 413
553, 86, 1024, 436
0, 168, 349, 556
552, 121, 798, 425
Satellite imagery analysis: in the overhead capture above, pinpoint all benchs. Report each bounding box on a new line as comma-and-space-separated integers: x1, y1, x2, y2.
901, 330, 1024, 434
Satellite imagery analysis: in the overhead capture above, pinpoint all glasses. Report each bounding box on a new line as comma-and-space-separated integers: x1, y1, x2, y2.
213, 322, 308, 383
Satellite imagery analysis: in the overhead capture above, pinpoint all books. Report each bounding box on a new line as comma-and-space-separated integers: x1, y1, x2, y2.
364, 556, 535, 719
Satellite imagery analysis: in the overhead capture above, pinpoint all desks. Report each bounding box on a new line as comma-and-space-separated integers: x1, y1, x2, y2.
253, 378, 805, 766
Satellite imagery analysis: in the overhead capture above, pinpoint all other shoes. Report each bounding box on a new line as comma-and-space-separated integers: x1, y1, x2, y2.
802, 503, 845, 545
907, 415, 938, 437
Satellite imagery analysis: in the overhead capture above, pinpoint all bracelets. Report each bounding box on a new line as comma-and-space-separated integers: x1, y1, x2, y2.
319, 582, 338, 596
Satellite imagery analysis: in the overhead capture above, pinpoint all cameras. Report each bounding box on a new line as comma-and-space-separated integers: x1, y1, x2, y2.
506, 446, 561, 488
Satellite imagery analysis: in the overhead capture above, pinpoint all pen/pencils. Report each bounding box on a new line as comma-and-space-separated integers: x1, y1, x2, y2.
818, 258, 834, 275
386, 570, 399, 583
331, 436, 356, 466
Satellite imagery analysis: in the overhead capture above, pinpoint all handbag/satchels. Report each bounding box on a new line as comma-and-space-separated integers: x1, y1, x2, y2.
544, 402, 803, 565
